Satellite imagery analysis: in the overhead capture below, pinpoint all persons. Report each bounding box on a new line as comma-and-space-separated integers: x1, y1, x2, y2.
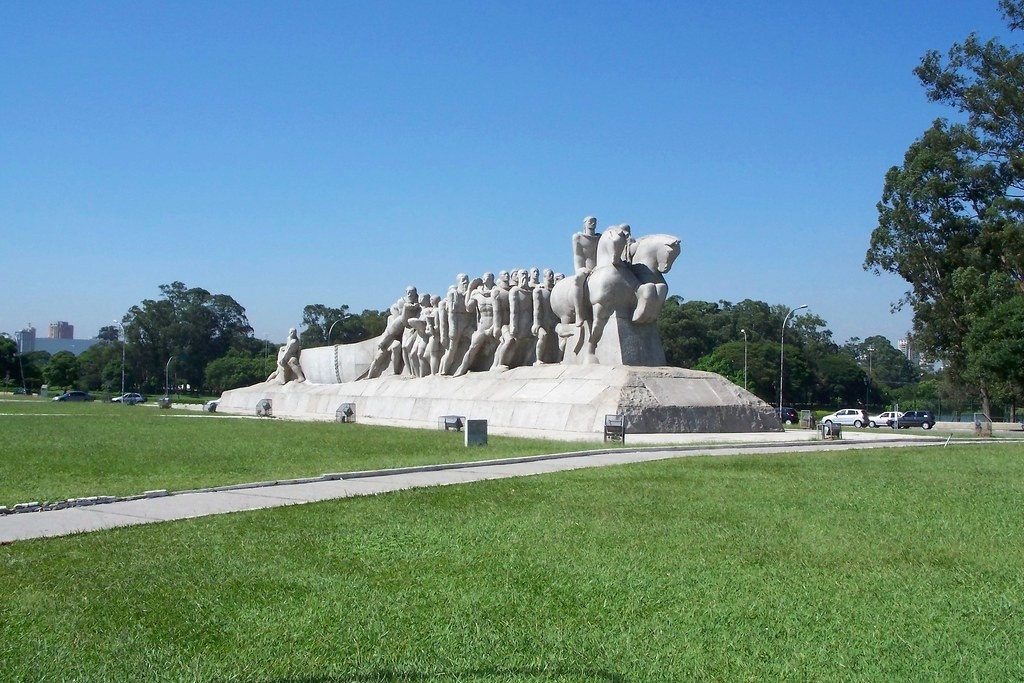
266, 216, 635, 385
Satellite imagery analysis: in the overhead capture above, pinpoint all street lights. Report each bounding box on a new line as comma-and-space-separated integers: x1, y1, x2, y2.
327, 314, 351, 346
741, 328, 748, 390
779, 304, 808, 425
112, 319, 125, 404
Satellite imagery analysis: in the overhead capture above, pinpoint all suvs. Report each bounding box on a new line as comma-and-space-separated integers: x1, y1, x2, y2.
774, 406, 799, 426
13, 388, 33, 395
868, 411, 905, 428
889, 410, 936, 430
821, 409, 870, 428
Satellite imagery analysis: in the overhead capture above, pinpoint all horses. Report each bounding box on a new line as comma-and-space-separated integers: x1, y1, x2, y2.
549, 224, 680, 367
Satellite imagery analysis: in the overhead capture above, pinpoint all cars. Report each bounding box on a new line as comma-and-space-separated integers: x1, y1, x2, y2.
111, 393, 144, 404
51, 391, 94, 403
